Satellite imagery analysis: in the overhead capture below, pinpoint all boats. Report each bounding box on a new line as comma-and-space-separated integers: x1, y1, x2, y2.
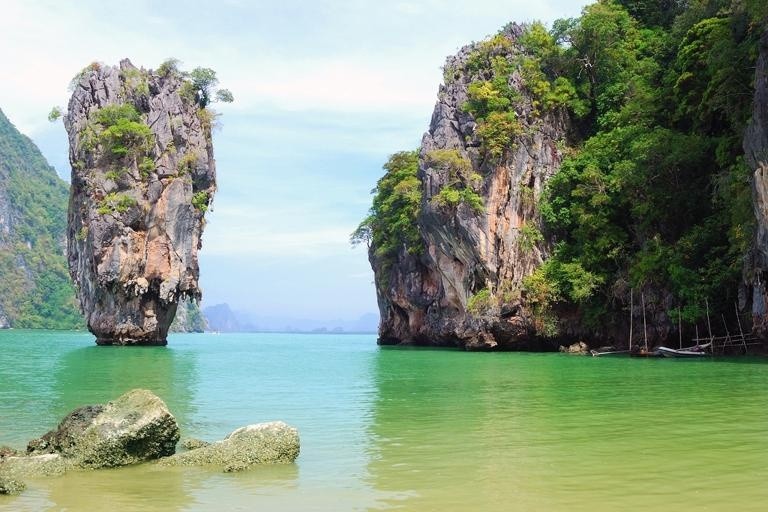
658, 346, 710, 359
